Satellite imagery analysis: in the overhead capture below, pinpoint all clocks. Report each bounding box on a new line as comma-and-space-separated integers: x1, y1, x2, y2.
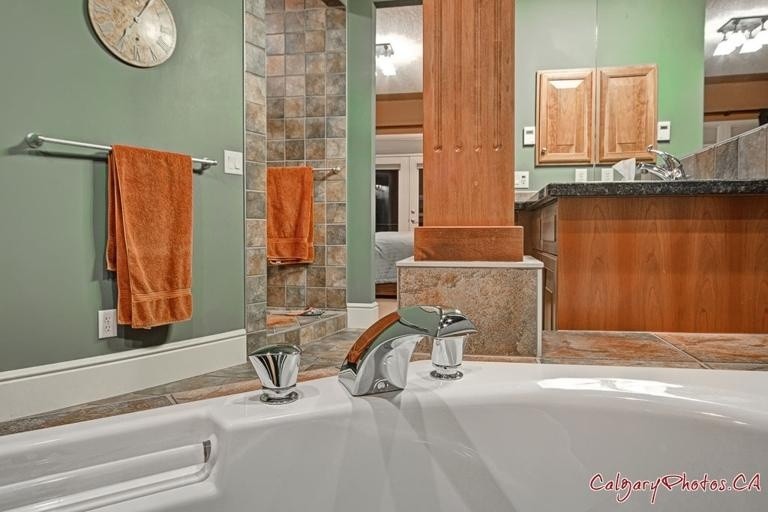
85, 0, 178, 69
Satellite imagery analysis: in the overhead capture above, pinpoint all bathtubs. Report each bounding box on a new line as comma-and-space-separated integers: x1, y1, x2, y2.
0, 368, 767, 511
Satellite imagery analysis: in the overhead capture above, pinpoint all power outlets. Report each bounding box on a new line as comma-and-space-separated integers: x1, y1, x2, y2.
96, 308, 119, 340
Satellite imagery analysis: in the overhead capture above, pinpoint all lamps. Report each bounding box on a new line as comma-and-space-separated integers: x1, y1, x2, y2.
711, 13, 768, 59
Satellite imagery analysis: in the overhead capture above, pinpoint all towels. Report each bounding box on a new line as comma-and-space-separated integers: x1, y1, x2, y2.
104, 141, 193, 329
267, 165, 315, 266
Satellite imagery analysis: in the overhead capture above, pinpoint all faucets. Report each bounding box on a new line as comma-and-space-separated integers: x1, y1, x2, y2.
636, 163, 674, 181
337, 304, 477, 397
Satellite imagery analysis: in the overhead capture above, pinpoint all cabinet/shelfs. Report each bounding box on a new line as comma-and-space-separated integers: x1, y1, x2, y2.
530, 201, 557, 332
594, 64, 659, 167
534, 68, 594, 166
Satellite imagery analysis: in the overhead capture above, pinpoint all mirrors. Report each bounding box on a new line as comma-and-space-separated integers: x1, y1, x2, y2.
595, 0, 768, 183
